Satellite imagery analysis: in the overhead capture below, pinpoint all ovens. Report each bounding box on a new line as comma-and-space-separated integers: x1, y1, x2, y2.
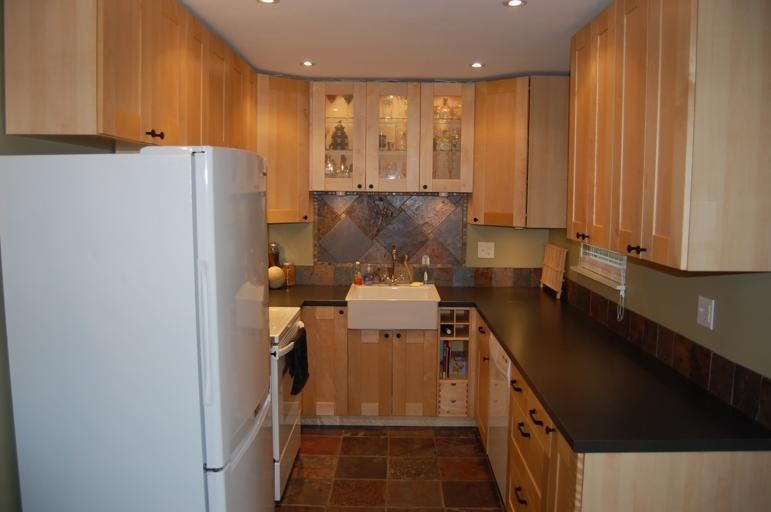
270, 317, 306, 505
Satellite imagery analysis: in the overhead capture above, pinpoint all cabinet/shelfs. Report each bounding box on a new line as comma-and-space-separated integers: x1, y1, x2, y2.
528, 71, 567, 230
0, 0, 185, 148
467, 75, 528, 228
346, 330, 437, 416
610, 0, 769, 275
185, 10, 255, 152
311, 79, 476, 194
302, 304, 347, 416
438, 307, 475, 419
255, 74, 311, 224
506, 368, 770, 512
564, 1, 616, 252
475, 311, 491, 453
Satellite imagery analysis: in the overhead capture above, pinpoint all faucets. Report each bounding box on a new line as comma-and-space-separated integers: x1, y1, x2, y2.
391, 249, 397, 283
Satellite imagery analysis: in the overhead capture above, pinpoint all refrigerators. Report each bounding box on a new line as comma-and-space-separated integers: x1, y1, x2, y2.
0, 142, 286, 512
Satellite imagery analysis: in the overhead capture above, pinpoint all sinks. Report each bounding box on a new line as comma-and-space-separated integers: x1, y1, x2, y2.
344, 282, 441, 330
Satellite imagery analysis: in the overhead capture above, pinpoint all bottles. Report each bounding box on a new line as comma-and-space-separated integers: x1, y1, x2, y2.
432, 134, 460, 152
330, 123, 348, 150
268, 242, 279, 267
438, 97, 452, 121
441, 324, 453, 336
364, 262, 374, 285
325, 152, 346, 178
354, 261, 362, 286
281, 262, 295, 286
399, 132, 407, 151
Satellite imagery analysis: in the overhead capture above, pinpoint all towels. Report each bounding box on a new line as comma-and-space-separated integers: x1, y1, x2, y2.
282, 327, 309, 395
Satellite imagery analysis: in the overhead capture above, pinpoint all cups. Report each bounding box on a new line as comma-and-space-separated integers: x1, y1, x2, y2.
381, 99, 393, 119
379, 135, 387, 150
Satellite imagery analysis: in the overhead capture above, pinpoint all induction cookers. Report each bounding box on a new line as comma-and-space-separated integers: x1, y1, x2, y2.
267, 304, 301, 344
